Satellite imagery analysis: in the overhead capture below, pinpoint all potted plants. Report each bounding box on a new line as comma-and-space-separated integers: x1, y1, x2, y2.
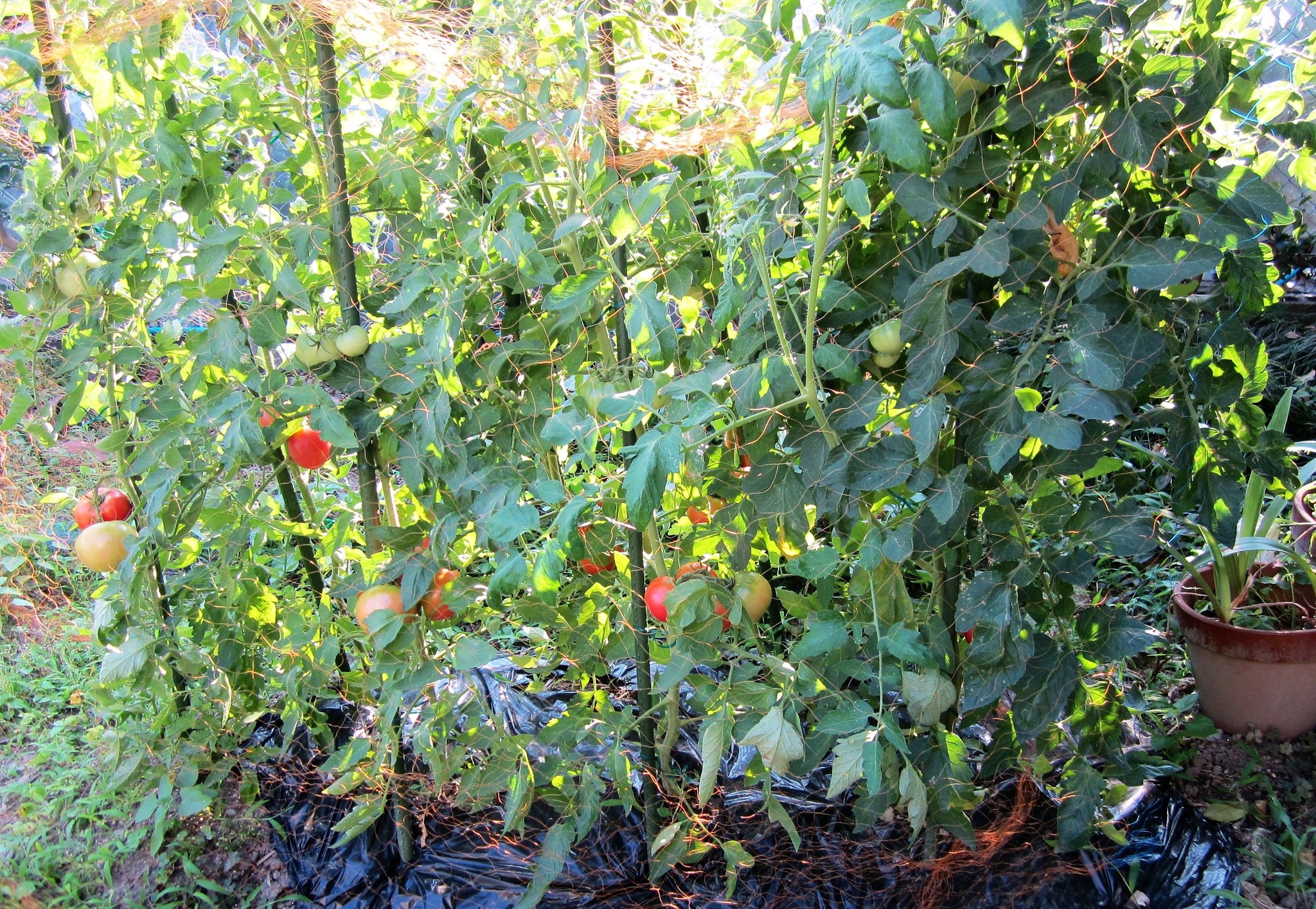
1144, 468, 1316, 739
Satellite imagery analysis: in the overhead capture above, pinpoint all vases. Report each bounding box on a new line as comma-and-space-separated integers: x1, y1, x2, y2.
1290, 480, 1316, 574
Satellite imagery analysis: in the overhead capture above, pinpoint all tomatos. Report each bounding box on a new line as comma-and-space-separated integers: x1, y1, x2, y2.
60, 223, 1078, 641
